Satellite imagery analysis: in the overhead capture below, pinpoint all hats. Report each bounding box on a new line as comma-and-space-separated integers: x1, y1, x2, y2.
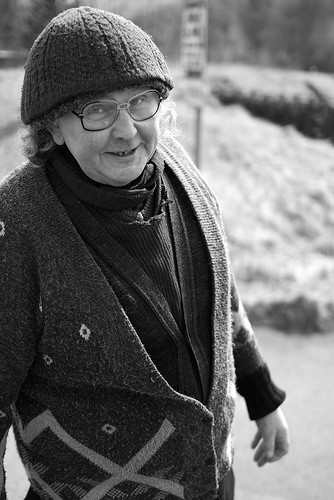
20, 6, 174, 125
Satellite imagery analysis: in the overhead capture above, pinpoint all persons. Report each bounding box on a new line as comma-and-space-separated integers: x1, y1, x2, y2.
0, 6, 289, 500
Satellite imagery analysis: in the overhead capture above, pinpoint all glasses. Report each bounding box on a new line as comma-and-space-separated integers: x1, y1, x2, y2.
72, 90, 163, 131
